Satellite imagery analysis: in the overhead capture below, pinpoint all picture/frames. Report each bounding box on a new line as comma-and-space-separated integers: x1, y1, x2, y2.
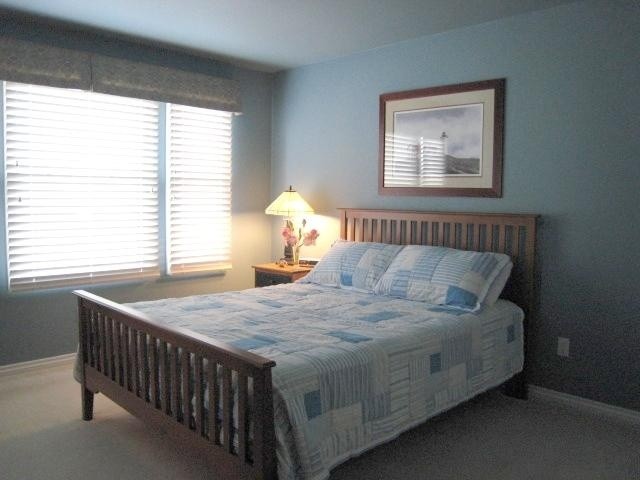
378, 78, 505, 198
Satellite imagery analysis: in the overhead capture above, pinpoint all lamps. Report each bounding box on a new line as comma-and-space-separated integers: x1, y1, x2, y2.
264, 185, 316, 265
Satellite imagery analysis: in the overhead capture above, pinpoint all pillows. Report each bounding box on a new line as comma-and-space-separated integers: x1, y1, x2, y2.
373, 246, 512, 315
294, 238, 406, 295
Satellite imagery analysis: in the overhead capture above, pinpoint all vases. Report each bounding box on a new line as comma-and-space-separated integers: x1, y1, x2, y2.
292, 245, 300, 267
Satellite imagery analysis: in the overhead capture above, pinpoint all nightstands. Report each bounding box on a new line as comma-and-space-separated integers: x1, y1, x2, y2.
251, 262, 317, 288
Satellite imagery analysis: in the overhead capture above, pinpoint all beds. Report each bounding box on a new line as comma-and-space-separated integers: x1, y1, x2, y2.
72, 209, 539, 480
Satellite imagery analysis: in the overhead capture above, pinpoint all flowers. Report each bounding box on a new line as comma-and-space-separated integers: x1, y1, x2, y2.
282, 220, 319, 247
